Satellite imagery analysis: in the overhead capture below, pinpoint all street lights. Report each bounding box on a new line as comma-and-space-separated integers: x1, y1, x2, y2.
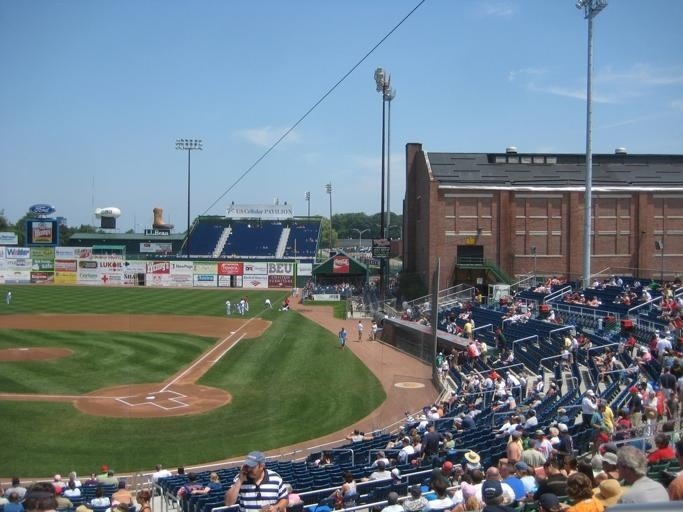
324, 181, 333, 251
352, 227, 372, 247
174, 137, 205, 262
572, 1, 615, 272
305, 190, 310, 218
372, 68, 391, 297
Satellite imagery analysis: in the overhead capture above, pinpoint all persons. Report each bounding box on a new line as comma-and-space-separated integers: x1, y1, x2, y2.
264, 296, 290, 311
1, 409, 682, 511
398, 273, 682, 429
226, 296, 249, 316
7, 290, 12, 304
304, 281, 400, 349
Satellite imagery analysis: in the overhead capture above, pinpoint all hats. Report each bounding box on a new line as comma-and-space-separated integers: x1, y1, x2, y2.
240, 450, 266, 466
377, 329, 661, 512
